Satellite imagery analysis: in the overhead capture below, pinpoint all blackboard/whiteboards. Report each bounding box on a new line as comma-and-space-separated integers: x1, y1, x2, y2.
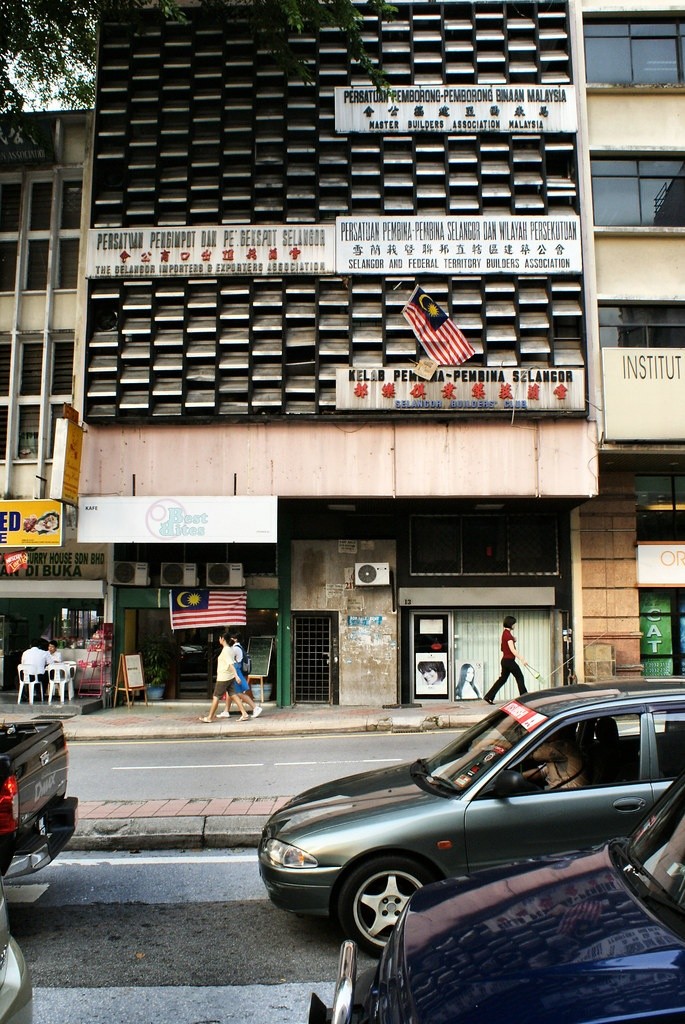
247, 635, 274, 676
115, 653, 145, 689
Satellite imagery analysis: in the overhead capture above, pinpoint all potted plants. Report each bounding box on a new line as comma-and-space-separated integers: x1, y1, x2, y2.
142, 630, 171, 698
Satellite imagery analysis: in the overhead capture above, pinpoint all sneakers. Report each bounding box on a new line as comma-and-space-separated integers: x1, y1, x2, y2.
251, 707, 263, 718
216, 712, 232, 718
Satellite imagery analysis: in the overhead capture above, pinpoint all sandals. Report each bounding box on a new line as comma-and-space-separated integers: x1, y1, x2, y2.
199, 717, 213, 722
236, 714, 250, 722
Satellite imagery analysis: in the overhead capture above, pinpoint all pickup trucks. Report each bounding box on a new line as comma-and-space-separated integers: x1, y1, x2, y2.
0, 719, 79, 902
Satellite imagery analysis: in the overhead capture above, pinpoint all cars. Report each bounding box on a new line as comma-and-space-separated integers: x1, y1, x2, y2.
309, 775, 684, 1024
180, 645, 207, 668
257, 677, 685, 958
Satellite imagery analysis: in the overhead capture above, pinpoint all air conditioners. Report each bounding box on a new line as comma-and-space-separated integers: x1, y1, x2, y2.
111, 561, 150, 586
160, 562, 197, 587
206, 562, 244, 587
355, 562, 390, 586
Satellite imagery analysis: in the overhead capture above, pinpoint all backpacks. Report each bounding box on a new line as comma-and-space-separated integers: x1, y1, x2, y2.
234, 645, 251, 676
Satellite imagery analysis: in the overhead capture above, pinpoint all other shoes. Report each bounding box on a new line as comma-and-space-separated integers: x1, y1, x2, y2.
484, 696, 494, 705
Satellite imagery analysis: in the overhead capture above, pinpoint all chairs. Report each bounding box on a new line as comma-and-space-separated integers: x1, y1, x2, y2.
18, 661, 76, 704
578, 717, 625, 785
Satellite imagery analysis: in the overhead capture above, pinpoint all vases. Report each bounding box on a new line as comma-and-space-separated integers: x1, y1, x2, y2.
251, 684, 272, 699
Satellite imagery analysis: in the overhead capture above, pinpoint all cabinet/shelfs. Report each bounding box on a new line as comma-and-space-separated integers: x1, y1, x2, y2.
78, 623, 113, 698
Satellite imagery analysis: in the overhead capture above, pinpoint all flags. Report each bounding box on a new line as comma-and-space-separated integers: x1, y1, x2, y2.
400, 283, 476, 365
169, 589, 248, 630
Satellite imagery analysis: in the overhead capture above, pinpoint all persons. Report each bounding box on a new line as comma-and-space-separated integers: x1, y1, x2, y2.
199, 630, 262, 723
483, 616, 529, 705
472, 725, 591, 791
417, 661, 446, 694
455, 664, 480, 699
21, 638, 62, 702
